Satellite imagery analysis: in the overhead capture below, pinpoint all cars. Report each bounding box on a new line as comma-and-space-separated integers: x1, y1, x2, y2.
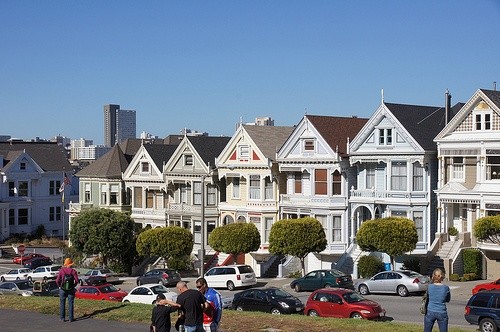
32, 281, 77, 297
356, 270, 430, 297
472, 279, 500, 296
304, 287, 386, 321
77, 268, 119, 286
213, 289, 232, 310
121, 284, 178, 306
75, 283, 128, 302
290, 269, 353, 292
24, 257, 52, 270
13, 253, 50, 265
0, 280, 36, 297
232, 287, 304, 315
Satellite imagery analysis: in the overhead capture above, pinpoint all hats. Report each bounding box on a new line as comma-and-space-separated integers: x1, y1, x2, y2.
64, 258, 74, 265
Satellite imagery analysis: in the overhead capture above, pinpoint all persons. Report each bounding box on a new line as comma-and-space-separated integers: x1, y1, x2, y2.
151, 293, 181, 332
424, 268, 450, 332
196, 278, 222, 332
176, 281, 210, 332
56, 258, 79, 323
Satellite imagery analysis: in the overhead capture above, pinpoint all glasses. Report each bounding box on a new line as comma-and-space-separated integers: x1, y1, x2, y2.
197, 284, 204, 288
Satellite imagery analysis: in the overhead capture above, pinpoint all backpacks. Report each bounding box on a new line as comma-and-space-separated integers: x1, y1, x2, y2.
61, 268, 74, 291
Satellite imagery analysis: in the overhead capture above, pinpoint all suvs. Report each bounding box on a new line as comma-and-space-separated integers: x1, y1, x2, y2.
464, 289, 500, 332
0, 268, 31, 282
27, 265, 62, 281
136, 269, 181, 288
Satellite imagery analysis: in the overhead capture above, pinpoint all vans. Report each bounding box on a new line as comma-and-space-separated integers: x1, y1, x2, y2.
198, 264, 257, 291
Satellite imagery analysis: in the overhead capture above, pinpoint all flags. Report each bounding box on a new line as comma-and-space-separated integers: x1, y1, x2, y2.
64, 173, 72, 185
59, 183, 65, 192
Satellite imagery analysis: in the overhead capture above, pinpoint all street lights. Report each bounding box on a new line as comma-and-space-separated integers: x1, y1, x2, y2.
201, 166, 235, 278
